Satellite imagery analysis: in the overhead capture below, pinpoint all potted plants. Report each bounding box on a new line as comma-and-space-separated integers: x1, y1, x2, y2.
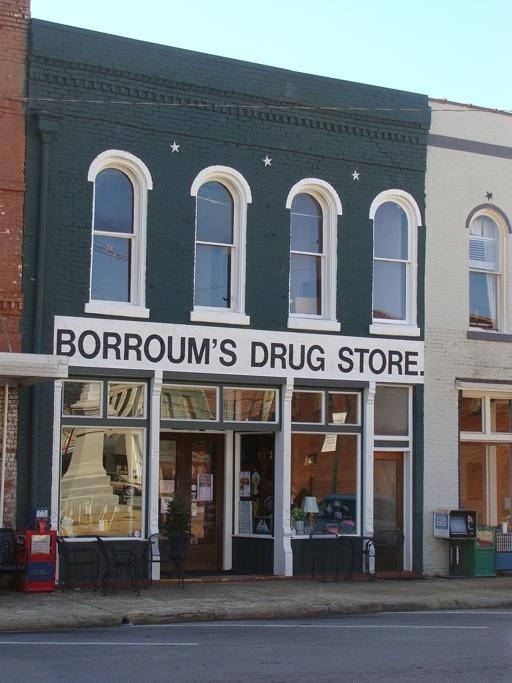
160, 491, 193, 559
292, 505, 308, 530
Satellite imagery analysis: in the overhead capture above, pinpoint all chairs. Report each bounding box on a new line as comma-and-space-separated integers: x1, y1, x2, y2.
54, 534, 194, 596
1, 527, 29, 595
306, 528, 406, 582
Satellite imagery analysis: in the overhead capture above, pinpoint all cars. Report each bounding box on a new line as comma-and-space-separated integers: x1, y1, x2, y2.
312, 494, 396, 574
106, 472, 142, 496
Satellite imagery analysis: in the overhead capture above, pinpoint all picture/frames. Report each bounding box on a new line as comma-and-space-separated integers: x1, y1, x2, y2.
240, 470, 254, 500
466, 462, 484, 501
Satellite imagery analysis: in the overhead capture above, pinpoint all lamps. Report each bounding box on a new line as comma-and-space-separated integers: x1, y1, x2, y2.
301, 496, 319, 531
